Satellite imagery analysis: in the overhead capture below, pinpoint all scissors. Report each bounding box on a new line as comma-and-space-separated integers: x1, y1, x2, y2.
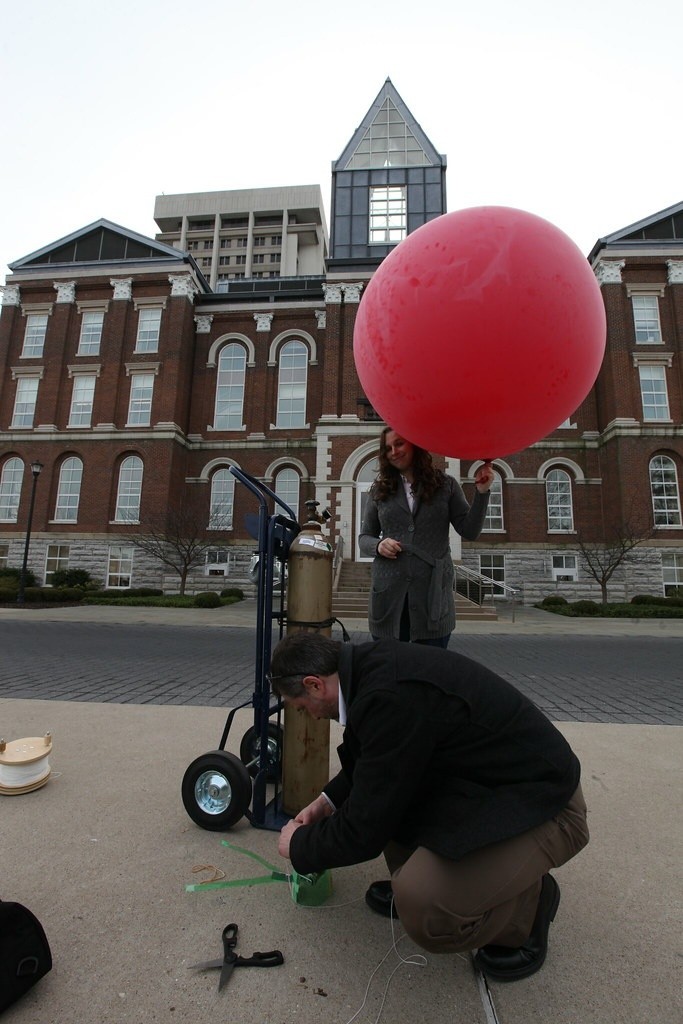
186, 922, 285, 993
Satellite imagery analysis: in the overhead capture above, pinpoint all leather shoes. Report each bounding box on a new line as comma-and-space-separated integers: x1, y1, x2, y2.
367, 879, 400, 920
475, 872, 561, 981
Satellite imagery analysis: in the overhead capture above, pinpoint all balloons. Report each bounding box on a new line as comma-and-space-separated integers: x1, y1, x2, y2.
352, 208, 607, 461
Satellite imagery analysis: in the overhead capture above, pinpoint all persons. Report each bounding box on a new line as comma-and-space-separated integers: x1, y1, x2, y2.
270, 633, 590, 981
359, 426, 494, 648
